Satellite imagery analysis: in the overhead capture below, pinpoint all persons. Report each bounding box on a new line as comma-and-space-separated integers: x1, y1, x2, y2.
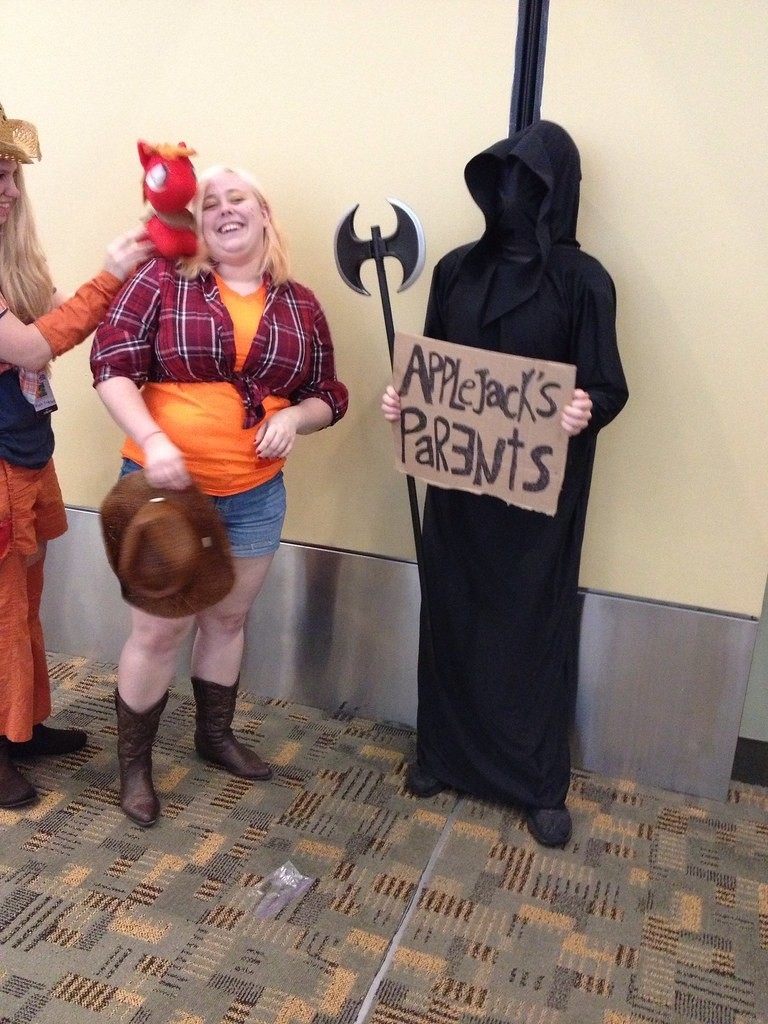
1, 103, 164, 809
382, 121, 631, 848
89, 166, 348, 829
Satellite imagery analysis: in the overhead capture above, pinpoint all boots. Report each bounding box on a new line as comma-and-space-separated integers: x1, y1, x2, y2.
113, 686, 169, 828
190, 671, 273, 779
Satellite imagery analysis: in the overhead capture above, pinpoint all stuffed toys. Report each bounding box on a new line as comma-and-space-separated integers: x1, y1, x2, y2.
138, 139, 198, 262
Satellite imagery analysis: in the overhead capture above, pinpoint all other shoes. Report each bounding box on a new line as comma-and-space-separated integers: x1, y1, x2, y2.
30, 724, 88, 756
0, 754, 38, 808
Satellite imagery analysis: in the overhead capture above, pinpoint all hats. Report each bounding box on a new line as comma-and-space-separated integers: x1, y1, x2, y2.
1, 103, 42, 165
98, 467, 236, 620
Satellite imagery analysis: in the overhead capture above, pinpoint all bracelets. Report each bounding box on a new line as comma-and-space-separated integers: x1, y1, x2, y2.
140, 429, 167, 446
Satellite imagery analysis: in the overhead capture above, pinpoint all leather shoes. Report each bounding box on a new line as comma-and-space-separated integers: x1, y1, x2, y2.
404, 762, 450, 797
524, 802, 574, 849
